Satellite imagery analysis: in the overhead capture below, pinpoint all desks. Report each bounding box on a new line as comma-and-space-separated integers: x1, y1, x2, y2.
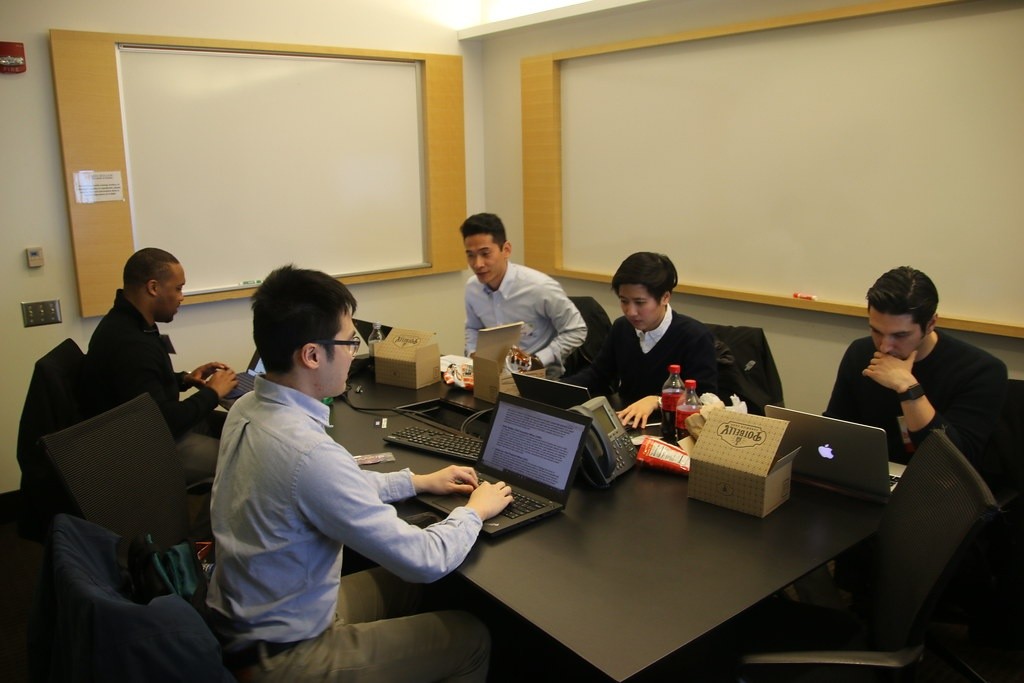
206, 355, 882, 683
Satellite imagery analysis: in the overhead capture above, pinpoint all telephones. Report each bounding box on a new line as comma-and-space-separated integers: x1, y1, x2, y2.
566, 395, 639, 486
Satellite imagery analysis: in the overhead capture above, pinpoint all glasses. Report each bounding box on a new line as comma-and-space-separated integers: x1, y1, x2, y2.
310, 337, 361, 357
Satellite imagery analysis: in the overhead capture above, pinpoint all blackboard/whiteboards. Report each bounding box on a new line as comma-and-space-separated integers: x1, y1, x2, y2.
519, 0, 1024, 343
45, 28, 467, 318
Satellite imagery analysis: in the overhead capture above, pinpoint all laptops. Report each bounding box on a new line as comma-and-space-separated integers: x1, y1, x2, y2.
765, 405, 908, 501
511, 372, 634, 432
414, 392, 593, 538
220, 349, 266, 400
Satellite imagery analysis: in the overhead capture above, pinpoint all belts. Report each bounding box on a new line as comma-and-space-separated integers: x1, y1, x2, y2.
223, 640, 302, 670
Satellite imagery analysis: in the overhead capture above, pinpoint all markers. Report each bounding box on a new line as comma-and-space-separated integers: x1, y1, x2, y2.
793, 293, 818, 301
239, 280, 263, 286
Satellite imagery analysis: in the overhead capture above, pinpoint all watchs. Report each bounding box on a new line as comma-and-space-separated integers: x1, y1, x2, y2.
897, 383, 924, 402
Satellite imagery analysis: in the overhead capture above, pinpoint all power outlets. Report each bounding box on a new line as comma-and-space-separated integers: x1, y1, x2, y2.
21, 299, 62, 328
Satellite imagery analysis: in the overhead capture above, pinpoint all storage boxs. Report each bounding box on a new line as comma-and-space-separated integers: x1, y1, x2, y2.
474, 321, 546, 402
374, 327, 441, 389
688, 406, 801, 519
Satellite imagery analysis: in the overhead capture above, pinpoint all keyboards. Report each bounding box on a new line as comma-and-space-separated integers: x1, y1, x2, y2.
383, 426, 483, 462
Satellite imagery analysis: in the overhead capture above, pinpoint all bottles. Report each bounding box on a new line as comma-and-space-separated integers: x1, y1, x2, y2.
368, 322, 386, 372
661, 365, 685, 445
675, 380, 702, 446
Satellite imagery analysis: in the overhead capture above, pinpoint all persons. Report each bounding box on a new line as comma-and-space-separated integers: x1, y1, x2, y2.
557, 251, 719, 430
208, 269, 514, 683
820, 266, 1008, 476
86, 247, 238, 542
459, 213, 588, 372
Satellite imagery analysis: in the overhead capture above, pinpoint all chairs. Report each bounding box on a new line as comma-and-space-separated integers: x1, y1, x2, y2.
40, 391, 212, 569
704, 323, 785, 417
561, 297, 614, 373
983, 380, 1024, 508
35, 338, 86, 440
743, 428, 1000, 666
47, 515, 231, 683
